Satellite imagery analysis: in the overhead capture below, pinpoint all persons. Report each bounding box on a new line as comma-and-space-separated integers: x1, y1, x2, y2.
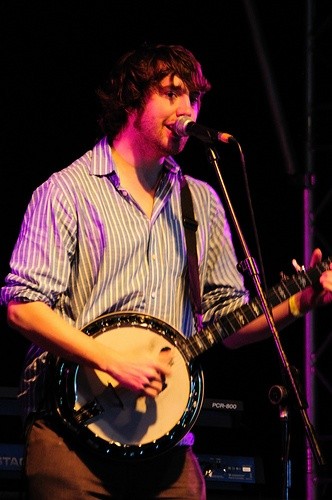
2, 35, 330, 500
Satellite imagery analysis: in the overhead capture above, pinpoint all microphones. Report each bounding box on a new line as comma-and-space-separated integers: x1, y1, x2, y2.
174, 116, 236, 143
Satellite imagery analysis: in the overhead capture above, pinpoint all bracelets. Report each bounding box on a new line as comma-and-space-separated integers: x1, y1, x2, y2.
288, 293, 304, 320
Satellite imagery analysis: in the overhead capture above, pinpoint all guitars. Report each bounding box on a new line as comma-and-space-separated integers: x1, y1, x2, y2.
58, 256, 332, 460
